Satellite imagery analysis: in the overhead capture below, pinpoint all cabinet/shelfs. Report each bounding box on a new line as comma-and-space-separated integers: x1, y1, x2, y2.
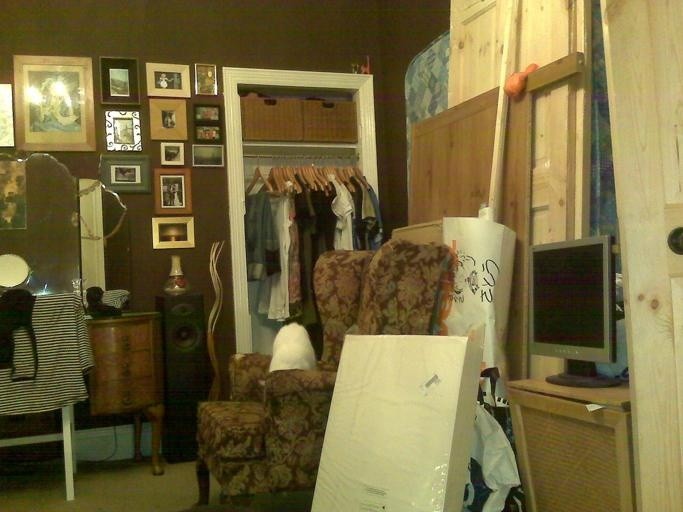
506, 378, 638, 511
81, 310, 167, 477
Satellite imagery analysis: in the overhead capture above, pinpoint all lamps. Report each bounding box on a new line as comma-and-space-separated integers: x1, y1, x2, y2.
163, 255, 190, 295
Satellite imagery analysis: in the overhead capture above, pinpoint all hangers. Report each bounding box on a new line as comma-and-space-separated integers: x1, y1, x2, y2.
245, 153, 372, 197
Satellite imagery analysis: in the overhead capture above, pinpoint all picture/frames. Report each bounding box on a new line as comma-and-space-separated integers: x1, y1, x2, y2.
14, 54, 94, 151
98, 55, 224, 250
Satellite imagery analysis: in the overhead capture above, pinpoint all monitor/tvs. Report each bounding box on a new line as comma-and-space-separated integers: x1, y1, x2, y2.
526, 235, 622, 388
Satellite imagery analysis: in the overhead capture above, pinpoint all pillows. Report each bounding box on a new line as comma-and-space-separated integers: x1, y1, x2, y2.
267, 323, 317, 373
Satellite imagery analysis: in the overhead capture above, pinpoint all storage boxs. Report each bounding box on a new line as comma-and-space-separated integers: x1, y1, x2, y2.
240, 95, 357, 143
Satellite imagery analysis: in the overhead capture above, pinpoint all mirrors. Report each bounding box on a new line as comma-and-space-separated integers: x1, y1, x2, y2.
0, 152, 106, 306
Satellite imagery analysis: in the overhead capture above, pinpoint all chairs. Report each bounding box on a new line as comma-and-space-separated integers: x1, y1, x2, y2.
195, 238, 449, 512
0, 294, 78, 500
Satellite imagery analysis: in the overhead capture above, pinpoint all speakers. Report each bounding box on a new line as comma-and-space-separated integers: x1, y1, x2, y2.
155, 292, 211, 464
